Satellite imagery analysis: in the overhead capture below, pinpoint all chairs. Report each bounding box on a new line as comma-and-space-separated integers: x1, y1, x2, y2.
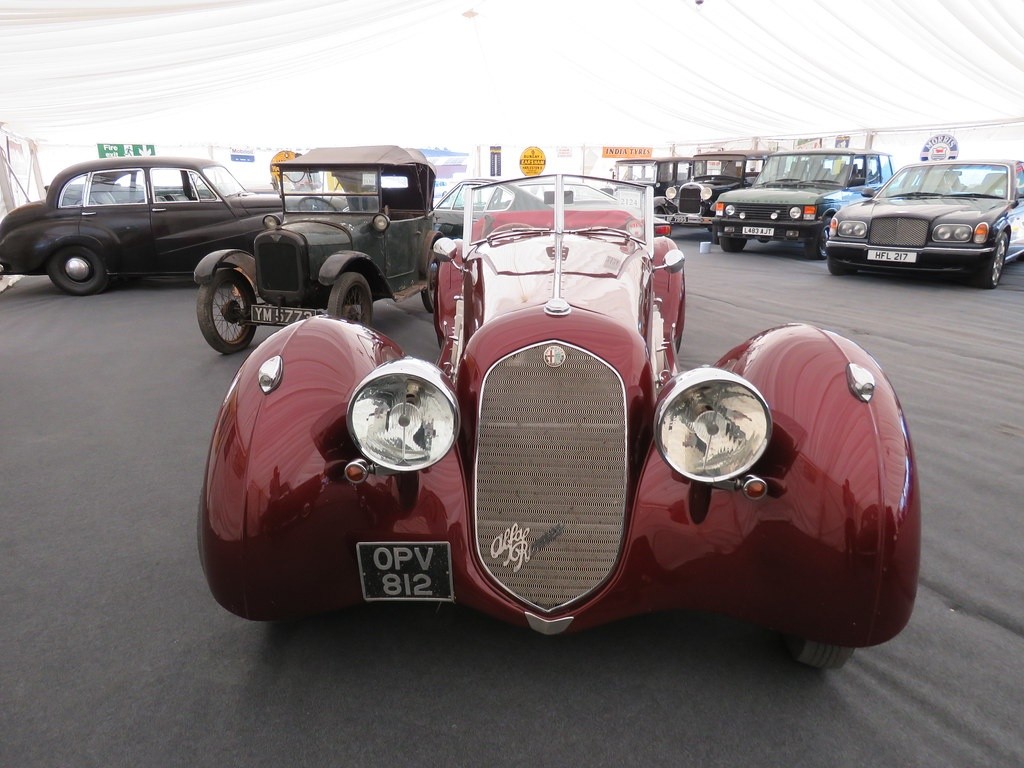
164, 194, 189, 202
858, 168, 872, 180
138, 196, 167, 202
812, 167, 832, 180
976, 173, 1007, 197
833, 164, 862, 186
917, 173, 953, 191
787, 160, 812, 182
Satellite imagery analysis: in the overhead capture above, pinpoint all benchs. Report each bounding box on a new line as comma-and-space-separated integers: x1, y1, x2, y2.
669, 172, 688, 180
63, 186, 131, 206
347, 187, 426, 215
472, 210, 641, 238
745, 172, 760, 178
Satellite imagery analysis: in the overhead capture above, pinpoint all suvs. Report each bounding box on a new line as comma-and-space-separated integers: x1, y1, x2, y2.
611, 149, 775, 244
716, 145, 897, 261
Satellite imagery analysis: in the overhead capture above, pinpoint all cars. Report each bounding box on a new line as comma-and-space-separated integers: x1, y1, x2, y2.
427, 175, 672, 249
0, 155, 351, 297
825, 156, 1024, 289
194, 143, 446, 355
198, 171, 923, 673
